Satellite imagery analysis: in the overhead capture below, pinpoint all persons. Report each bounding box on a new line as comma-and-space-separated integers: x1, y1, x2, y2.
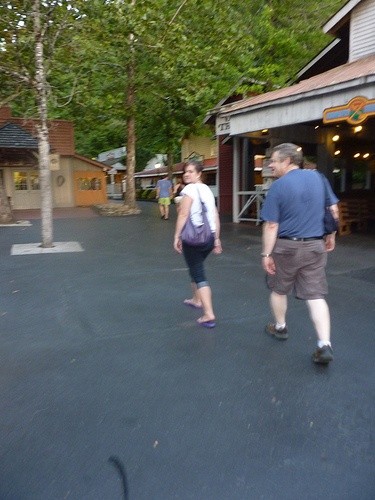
256, 143, 340, 363
174, 161, 222, 329
154, 179, 186, 220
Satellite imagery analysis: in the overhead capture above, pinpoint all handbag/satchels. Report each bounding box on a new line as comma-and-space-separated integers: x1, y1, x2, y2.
323, 206, 337, 234
177, 185, 211, 246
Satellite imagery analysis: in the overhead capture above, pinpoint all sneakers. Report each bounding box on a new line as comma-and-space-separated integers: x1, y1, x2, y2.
265, 321, 288, 339
311, 344, 334, 362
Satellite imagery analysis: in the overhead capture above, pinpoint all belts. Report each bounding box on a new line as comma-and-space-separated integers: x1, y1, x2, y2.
277, 235, 323, 241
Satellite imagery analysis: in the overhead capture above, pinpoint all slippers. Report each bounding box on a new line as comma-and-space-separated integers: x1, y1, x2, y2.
195, 316, 216, 328
184, 299, 202, 308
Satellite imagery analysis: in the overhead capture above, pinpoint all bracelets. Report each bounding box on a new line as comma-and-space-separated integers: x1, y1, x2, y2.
261, 254, 272, 257
